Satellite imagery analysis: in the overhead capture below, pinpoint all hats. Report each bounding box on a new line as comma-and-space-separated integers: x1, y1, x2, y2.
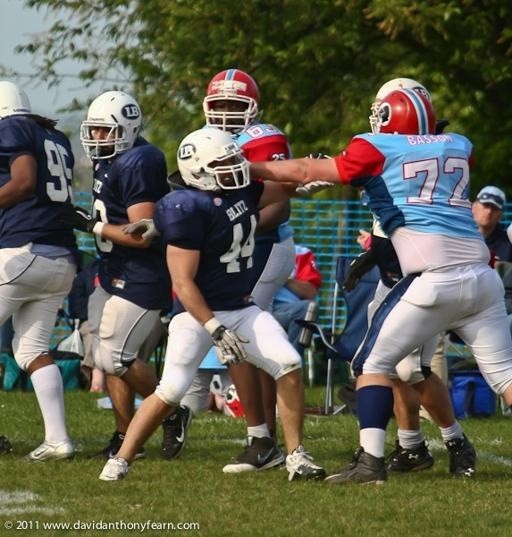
475, 185, 506, 211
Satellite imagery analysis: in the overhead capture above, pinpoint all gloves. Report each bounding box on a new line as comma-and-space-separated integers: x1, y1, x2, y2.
203, 315, 250, 368
120, 218, 162, 241
294, 181, 337, 199
58, 205, 99, 234
302, 152, 334, 160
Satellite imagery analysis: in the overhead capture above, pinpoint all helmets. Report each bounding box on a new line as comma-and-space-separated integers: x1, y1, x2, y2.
174, 127, 252, 193
236, 123, 293, 180
368, 76, 437, 137
202, 68, 261, 137
78, 89, 143, 162
0, 79, 32, 121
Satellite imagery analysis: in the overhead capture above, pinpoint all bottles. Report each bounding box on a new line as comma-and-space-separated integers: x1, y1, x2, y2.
297, 301, 317, 346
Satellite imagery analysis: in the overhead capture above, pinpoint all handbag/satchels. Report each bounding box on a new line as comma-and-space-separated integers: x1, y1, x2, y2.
448, 369, 495, 420
0, 348, 82, 393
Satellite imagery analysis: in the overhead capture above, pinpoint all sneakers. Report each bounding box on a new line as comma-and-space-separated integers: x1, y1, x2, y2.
87, 429, 147, 462
20, 436, 77, 463
285, 444, 326, 483
443, 431, 478, 481
322, 445, 389, 487
160, 403, 194, 462
221, 430, 285, 475
97, 455, 130, 483
384, 439, 436, 474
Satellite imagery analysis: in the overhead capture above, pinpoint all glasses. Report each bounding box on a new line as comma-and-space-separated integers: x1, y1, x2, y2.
478, 192, 504, 208
